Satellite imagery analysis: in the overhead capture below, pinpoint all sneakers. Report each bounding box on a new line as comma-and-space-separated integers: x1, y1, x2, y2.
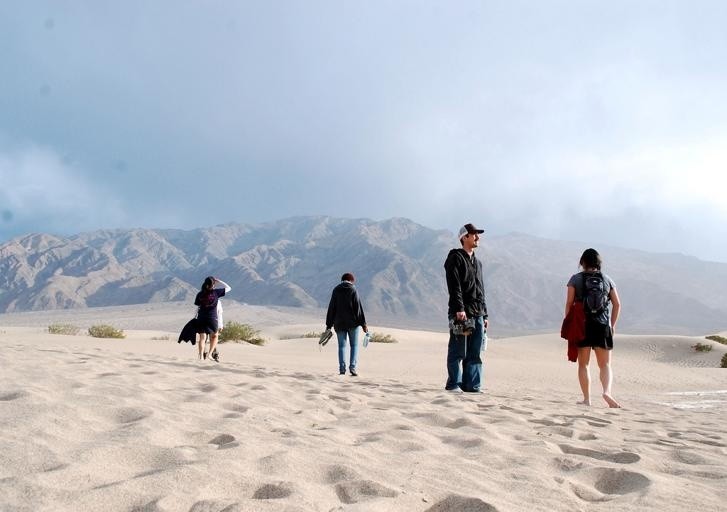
319, 329, 333, 346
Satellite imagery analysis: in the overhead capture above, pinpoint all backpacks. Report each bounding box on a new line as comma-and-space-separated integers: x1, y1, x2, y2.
579, 270, 607, 317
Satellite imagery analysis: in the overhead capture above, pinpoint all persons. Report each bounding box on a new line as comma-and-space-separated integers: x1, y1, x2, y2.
201, 299, 224, 363
325, 272, 369, 376
192, 276, 231, 361
443, 223, 488, 394
564, 248, 624, 409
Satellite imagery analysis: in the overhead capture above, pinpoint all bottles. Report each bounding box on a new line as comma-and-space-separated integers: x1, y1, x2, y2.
363, 333, 370, 348
482, 329, 487, 351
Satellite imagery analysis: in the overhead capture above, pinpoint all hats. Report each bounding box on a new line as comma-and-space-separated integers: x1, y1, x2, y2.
456, 223, 486, 240
